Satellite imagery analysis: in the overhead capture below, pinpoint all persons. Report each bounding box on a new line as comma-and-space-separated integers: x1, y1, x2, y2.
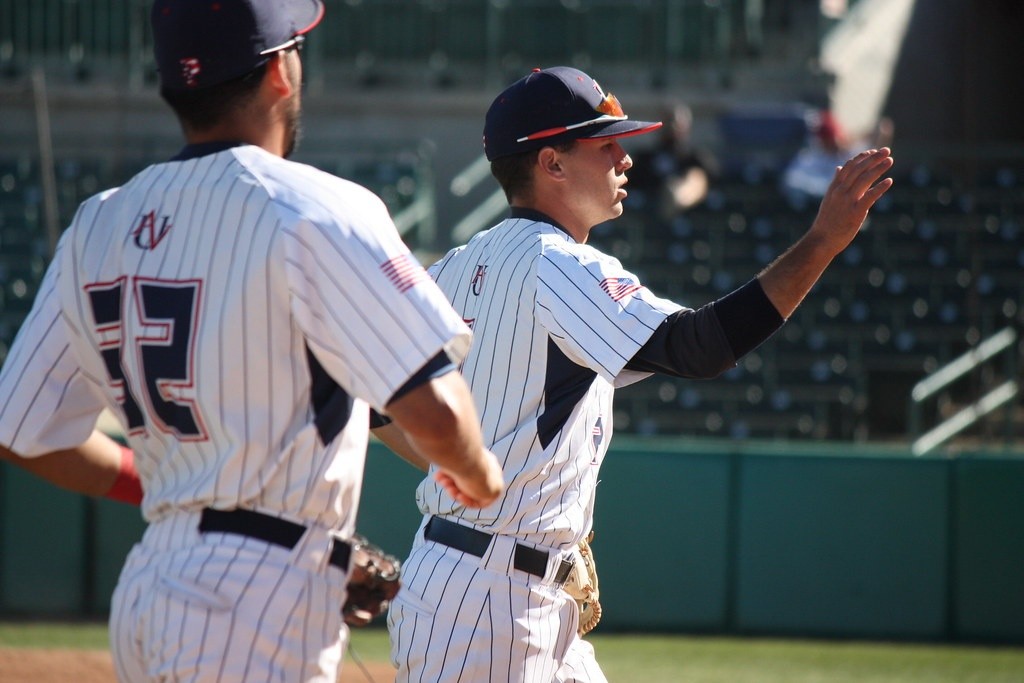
0, 0, 504, 683
370, 66, 893, 683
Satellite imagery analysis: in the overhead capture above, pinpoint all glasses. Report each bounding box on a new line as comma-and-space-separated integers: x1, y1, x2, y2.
516, 91, 628, 142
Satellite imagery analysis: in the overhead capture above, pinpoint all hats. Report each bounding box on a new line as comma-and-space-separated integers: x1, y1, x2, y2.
147, 1, 323, 58
483, 67, 663, 160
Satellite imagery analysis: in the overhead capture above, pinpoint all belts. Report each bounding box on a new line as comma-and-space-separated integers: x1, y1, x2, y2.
197, 505, 351, 571
422, 512, 576, 584
